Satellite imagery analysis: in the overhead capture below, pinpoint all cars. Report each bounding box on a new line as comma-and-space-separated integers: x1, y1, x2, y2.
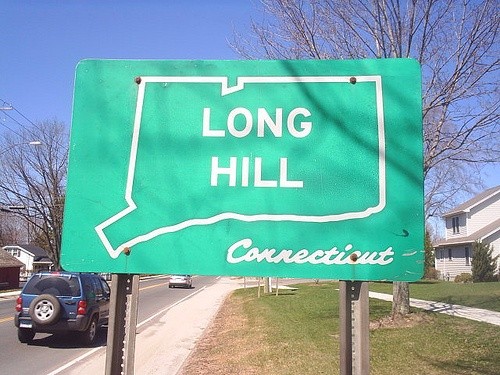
167, 275, 193, 288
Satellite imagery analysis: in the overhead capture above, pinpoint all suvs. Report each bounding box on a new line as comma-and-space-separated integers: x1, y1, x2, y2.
13, 272, 111, 346
